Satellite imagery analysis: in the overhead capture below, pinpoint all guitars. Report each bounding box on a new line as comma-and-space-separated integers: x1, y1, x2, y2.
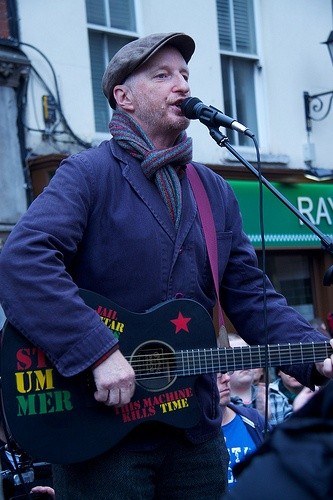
0, 286, 333, 469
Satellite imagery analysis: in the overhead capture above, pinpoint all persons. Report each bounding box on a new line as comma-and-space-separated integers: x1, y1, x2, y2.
215, 321, 333, 499
0, 32, 333, 499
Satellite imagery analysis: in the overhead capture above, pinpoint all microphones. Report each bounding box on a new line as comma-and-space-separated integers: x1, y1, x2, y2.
180, 96, 254, 138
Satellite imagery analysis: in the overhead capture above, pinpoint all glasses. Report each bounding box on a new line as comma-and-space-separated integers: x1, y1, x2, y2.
216, 370, 233, 378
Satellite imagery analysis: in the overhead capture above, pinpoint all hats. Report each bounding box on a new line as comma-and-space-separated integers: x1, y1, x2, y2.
101, 30, 196, 106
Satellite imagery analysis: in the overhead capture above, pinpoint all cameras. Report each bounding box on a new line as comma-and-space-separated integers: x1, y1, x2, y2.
9, 492, 53, 500
